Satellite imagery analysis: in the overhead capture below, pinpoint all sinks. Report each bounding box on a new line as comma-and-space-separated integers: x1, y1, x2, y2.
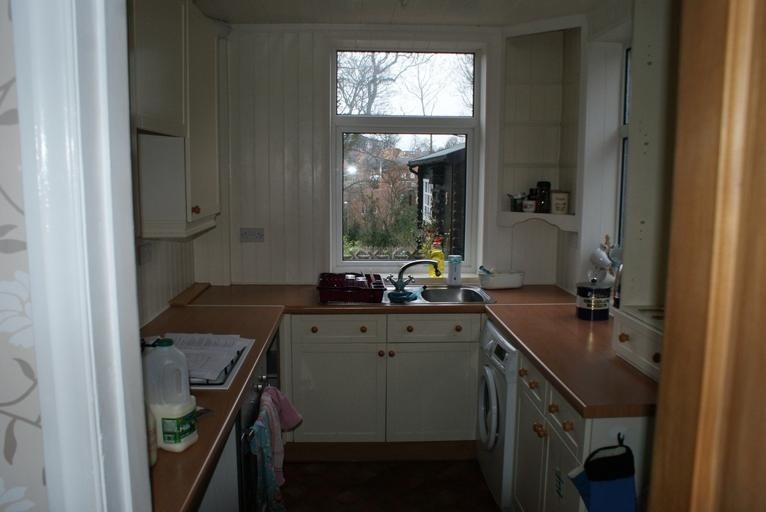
420, 285, 496, 304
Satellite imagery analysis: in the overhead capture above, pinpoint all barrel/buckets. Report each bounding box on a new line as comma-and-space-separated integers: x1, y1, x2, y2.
142, 338, 199, 454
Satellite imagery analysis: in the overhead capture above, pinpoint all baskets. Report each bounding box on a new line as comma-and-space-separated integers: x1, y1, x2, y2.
478, 271, 524, 289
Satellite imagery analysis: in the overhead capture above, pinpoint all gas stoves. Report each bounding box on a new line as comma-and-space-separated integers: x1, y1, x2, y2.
187, 338, 255, 391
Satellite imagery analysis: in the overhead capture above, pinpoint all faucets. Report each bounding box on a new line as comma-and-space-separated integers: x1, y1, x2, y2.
398, 260, 441, 281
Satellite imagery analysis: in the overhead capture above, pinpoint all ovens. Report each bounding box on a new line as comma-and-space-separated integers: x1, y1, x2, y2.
235, 331, 268, 512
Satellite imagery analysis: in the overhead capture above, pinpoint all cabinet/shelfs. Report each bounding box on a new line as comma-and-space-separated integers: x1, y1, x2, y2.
278, 314, 481, 441
510, 353, 652, 512
610, 3, 679, 385
130, 0, 221, 240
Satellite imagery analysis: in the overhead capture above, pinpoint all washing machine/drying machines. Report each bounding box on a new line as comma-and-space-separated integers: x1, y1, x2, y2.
474, 319, 520, 510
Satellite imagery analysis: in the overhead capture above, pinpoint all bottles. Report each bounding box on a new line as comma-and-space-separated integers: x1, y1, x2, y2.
446, 255, 462, 286
428, 236, 445, 277
526, 181, 569, 214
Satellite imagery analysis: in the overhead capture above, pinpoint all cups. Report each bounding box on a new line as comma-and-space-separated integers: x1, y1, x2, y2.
510, 199, 536, 213
576, 278, 611, 321
